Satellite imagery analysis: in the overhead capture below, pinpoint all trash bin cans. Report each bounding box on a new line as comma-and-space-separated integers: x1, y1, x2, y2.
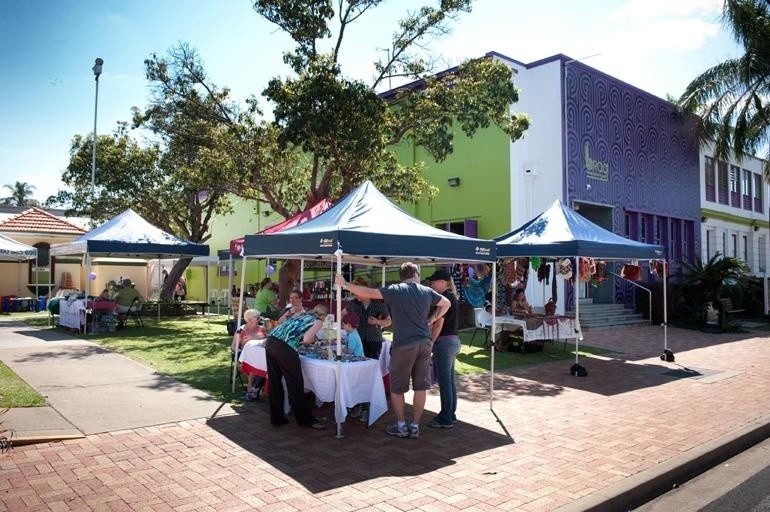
39, 295, 46, 311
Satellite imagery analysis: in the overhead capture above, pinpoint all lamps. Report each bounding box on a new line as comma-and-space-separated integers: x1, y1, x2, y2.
261, 211, 269, 217
448, 177, 460, 187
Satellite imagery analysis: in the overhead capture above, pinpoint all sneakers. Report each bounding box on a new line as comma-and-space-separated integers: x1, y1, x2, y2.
248, 386, 260, 400
307, 422, 326, 430
386, 421, 408, 437
408, 422, 420, 439
428, 413, 457, 428
350, 404, 368, 422
272, 418, 290, 427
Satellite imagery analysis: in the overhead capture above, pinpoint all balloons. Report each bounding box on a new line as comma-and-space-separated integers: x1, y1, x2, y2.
88, 272, 96, 280
266, 265, 274, 274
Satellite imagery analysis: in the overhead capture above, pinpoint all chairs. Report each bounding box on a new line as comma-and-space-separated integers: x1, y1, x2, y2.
468, 307, 492, 349
208, 288, 230, 307
231, 345, 247, 393
4, 283, 146, 332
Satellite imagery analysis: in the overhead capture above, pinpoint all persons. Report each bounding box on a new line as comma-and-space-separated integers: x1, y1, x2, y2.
232, 277, 392, 429
100, 270, 186, 329
512, 288, 529, 313
484, 291, 508, 316
335, 262, 451, 439
425, 270, 461, 428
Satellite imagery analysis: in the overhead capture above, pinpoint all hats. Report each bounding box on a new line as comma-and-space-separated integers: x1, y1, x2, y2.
425, 270, 450, 281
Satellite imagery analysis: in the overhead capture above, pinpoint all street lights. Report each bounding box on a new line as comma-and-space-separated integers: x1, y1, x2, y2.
82, 57, 105, 335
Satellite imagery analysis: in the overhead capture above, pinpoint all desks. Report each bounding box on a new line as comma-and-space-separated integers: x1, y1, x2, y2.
489, 317, 583, 357
236, 337, 388, 435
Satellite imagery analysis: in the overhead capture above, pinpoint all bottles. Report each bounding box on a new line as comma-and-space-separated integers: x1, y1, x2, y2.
311, 286, 335, 301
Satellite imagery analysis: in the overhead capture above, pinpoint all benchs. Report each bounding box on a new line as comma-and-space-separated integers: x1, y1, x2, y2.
718, 298, 746, 328
